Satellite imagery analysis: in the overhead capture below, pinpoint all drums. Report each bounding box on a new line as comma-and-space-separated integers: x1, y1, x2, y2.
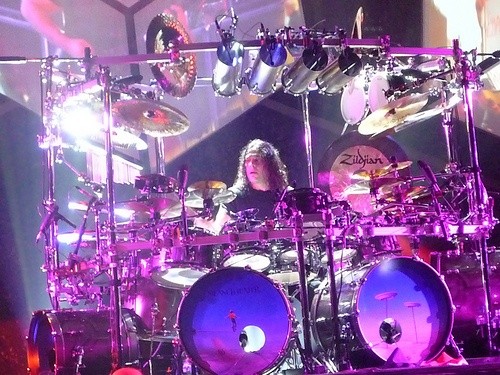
118, 187, 363, 344
24, 306, 140, 375
308, 256, 454, 368
173, 263, 297, 375
431, 247, 500, 357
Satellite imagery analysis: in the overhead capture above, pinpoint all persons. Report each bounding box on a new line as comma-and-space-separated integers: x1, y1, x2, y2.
214, 138, 295, 258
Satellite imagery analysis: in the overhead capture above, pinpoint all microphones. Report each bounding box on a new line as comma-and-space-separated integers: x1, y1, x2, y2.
177, 164, 188, 199
418, 160, 442, 197
239, 331, 248, 347
43, 203, 77, 228
75, 185, 102, 203
35, 205, 59, 244
273, 189, 287, 213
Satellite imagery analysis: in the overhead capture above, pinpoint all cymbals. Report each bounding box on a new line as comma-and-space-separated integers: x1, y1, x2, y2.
341, 161, 443, 203
58, 180, 239, 245
146, 13, 196, 98
359, 93, 444, 141
112, 99, 191, 137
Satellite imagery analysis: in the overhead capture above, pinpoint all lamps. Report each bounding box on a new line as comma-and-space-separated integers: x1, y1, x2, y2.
212, 15, 362, 98
